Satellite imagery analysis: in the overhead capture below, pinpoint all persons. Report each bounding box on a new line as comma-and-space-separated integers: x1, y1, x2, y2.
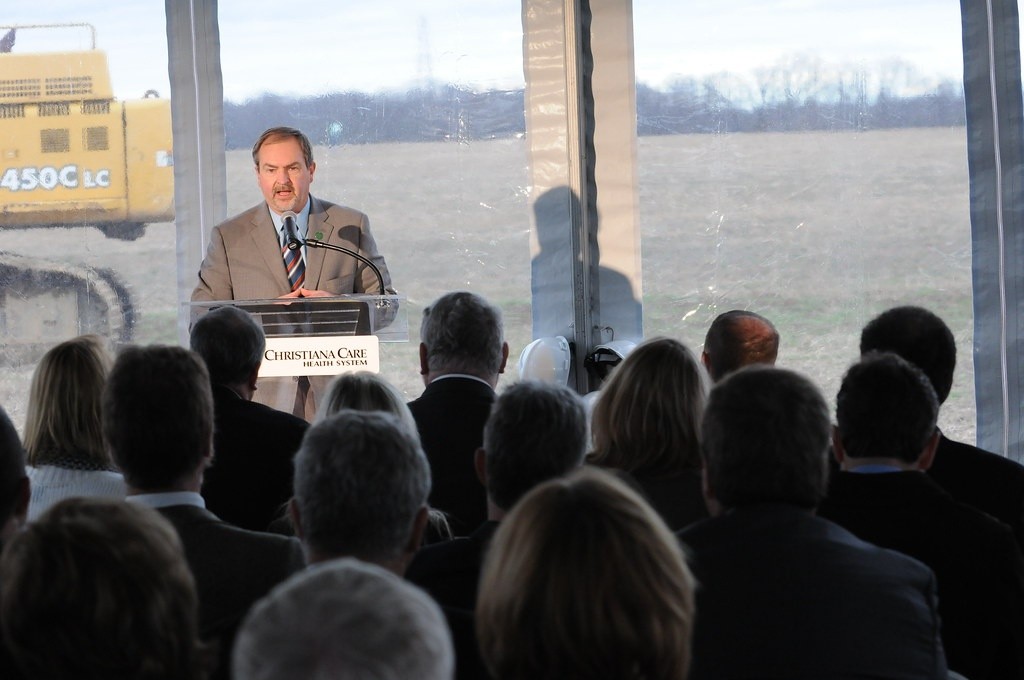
231, 561, 454, 680
319, 374, 454, 545
104, 345, 307, 644
22, 333, 128, 520
820, 352, 1024, 680
702, 310, 778, 386
0, 408, 31, 551
673, 365, 949, 680
407, 292, 508, 536
828, 305, 1024, 526
403, 379, 588, 613
189, 306, 309, 532
476, 467, 698, 680
191, 127, 397, 418
0, 495, 204, 680
286, 407, 493, 680
581, 336, 709, 530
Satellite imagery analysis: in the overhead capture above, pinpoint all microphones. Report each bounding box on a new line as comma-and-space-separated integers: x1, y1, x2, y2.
279, 211, 299, 249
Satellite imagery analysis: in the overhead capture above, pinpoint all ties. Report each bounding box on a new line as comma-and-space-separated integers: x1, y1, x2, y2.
283, 229, 306, 299
